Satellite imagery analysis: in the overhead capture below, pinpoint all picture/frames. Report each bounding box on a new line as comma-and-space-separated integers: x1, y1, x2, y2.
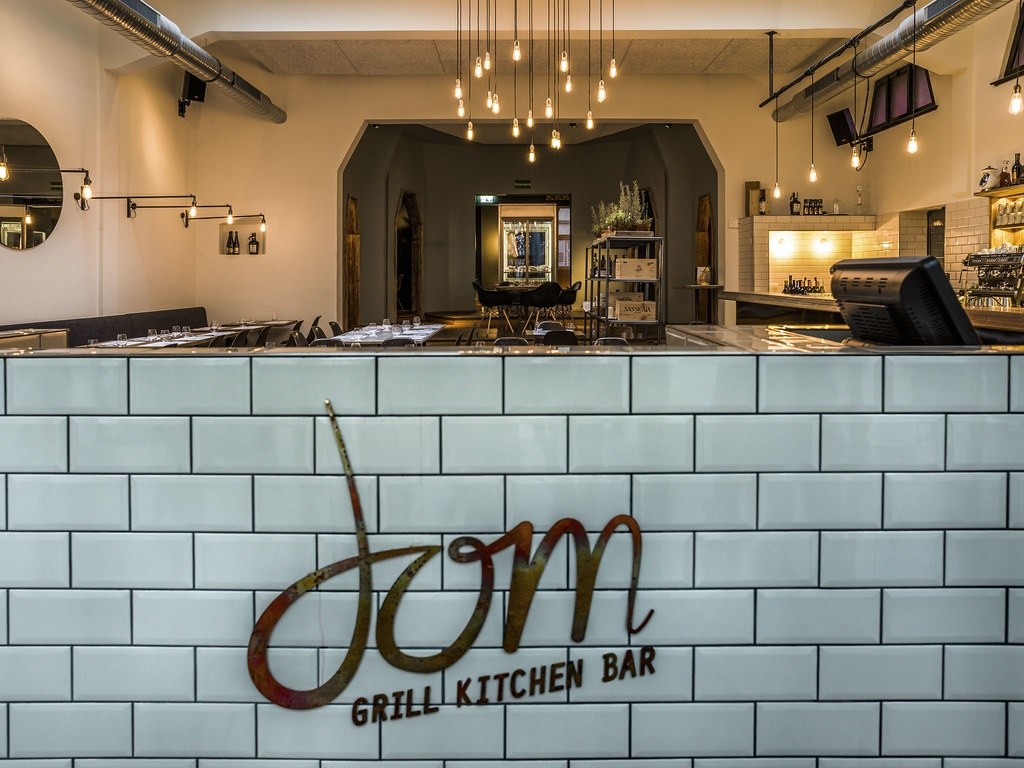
0, 217, 24, 251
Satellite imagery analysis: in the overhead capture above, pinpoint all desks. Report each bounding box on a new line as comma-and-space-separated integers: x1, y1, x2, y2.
75, 319, 447, 348
471, 327, 585, 342
497, 283, 542, 331
671, 284, 722, 325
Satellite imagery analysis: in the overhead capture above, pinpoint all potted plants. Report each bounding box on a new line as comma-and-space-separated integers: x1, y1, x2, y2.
591, 178, 655, 238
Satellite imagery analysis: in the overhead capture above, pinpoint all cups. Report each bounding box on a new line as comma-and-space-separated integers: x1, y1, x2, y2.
354, 315, 423, 343
87, 312, 279, 347
567, 322, 574, 331
995, 200, 1024, 226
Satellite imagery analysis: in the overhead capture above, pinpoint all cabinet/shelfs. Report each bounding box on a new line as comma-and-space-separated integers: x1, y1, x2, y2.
497, 204, 558, 282
584, 235, 664, 346
974, 183, 1024, 250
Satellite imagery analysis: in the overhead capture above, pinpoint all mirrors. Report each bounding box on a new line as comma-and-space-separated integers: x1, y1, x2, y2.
0, 117, 64, 251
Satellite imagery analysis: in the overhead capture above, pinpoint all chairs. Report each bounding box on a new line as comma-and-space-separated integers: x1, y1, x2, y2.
454, 321, 629, 346
472, 281, 582, 335
209, 315, 417, 347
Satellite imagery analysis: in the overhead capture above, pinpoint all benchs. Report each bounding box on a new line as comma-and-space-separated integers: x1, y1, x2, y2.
0, 307, 208, 351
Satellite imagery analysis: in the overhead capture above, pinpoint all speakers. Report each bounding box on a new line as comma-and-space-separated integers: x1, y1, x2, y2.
181, 70, 207, 102
826, 108, 858, 146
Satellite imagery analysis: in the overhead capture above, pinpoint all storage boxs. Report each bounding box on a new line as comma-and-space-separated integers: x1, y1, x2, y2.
599, 291, 656, 321
600, 326, 632, 339
612, 258, 658, 279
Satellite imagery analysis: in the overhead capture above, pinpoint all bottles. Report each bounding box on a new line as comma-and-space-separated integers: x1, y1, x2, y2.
998, 151, 1022, 186
759, 196, 766, 215
782, 275, 825, 295
789, 192, 823, 215
591, 252, 626, 277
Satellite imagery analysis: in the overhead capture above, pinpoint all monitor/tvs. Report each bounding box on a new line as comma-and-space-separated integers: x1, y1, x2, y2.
831, 255, 982, 346
